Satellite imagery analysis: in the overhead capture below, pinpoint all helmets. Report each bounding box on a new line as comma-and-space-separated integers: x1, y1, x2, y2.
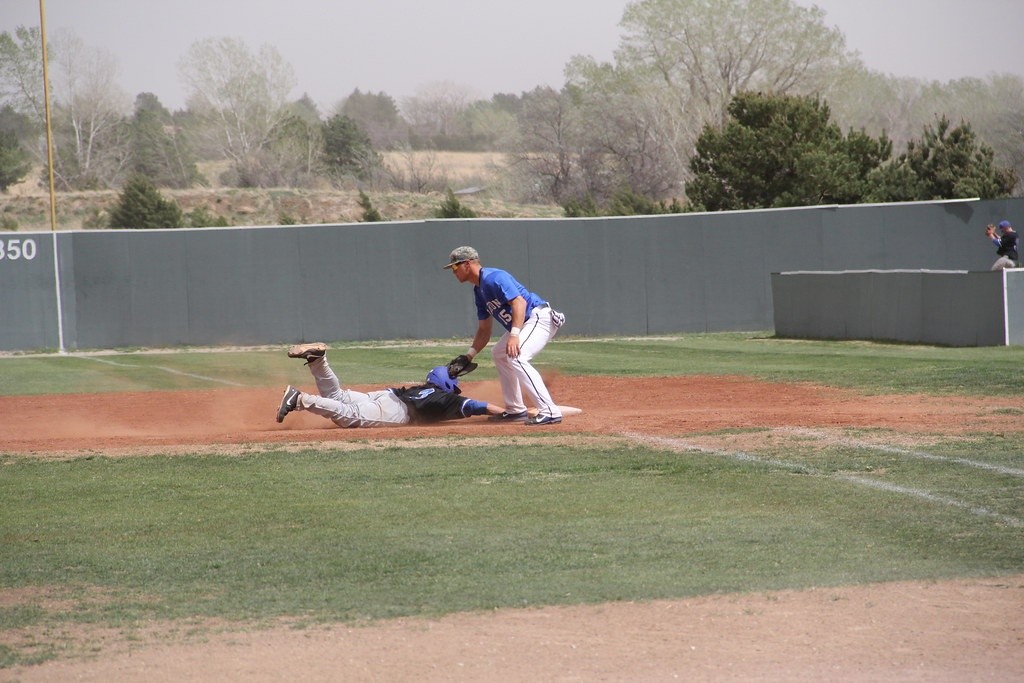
999, 221, 1011, 230
426, 366, 459, 394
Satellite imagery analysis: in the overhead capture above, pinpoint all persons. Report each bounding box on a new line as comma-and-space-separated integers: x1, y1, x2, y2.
444, 246, 565, 424
984, 220, 1019, 271
276, 341, 580, 428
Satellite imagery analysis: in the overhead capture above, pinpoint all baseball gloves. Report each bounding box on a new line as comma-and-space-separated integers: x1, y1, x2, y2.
446, 354, 478, 379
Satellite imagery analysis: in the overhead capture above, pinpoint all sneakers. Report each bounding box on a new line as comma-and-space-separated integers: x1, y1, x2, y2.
488, 411, 528, 423
524, 414, 561, 425
288, 342, 326, 363
277, 385, 301, 423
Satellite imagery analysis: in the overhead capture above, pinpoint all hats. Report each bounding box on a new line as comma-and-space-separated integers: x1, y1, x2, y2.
443, 246, 479, 269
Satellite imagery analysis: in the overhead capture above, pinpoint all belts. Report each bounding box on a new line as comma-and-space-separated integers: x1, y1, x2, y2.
537, 303, 549, 310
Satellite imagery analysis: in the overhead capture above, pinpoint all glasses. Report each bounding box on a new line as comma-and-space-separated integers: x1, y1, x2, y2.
451, 264, 461, 270
